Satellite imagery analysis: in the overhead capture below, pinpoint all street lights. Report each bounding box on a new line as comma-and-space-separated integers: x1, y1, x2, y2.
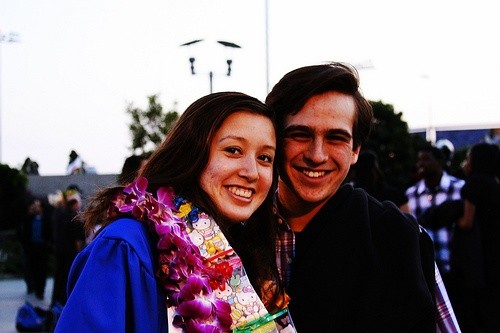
180, 36, 242, 95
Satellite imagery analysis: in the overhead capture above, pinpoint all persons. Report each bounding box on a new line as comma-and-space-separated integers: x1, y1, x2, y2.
51, 199, 87, 306
15, 91, 299, 333
435, 146, 466, 175
456, 141, 499, 289
241, 61, 462, 333
400, 146, 479, 332
18, 199, 55, 300
